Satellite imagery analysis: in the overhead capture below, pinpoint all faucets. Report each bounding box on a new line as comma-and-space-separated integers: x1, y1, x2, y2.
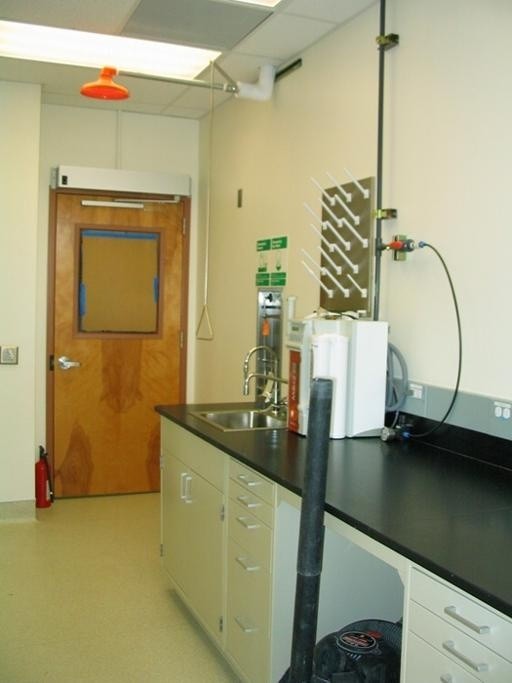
242, 372, 287, 396
243, 345, 280, 389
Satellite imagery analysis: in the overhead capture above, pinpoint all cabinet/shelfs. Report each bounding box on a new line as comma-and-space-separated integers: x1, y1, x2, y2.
156, 406, 226, 656
400, 557, 512, 683
227, 451, 399, 683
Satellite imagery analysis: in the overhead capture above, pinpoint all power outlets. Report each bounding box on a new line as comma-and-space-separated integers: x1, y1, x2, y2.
409, 385, 423, 400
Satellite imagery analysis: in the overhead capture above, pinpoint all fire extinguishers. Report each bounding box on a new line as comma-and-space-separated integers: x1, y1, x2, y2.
35, 445, 54, 508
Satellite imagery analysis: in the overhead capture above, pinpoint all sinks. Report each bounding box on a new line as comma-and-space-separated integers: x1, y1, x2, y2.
188, 408, 288, 433
